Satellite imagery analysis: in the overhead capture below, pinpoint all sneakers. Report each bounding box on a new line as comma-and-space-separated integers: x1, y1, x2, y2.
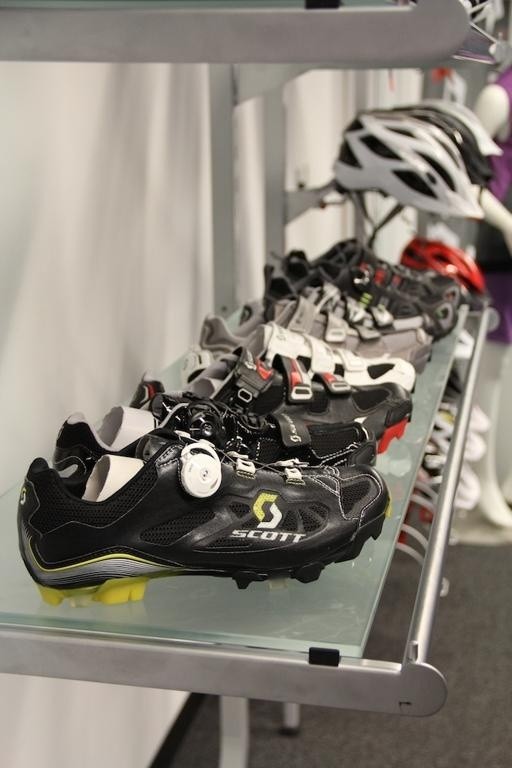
18, 428, 390, 608
52, 392, 377, 489
264, 250, 458, 338
200, 293, 433, 369
182, 313, 417, 394
317, 237, 460, 311
130, 347, 412, 454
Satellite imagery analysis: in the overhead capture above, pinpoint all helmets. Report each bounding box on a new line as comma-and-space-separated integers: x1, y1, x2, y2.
394, 99, 504, 184
403, 238, 488, 309
335, 109, 484, 221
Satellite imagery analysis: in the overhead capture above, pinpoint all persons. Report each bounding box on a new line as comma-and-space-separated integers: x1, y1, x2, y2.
470, 64, 512, 528
